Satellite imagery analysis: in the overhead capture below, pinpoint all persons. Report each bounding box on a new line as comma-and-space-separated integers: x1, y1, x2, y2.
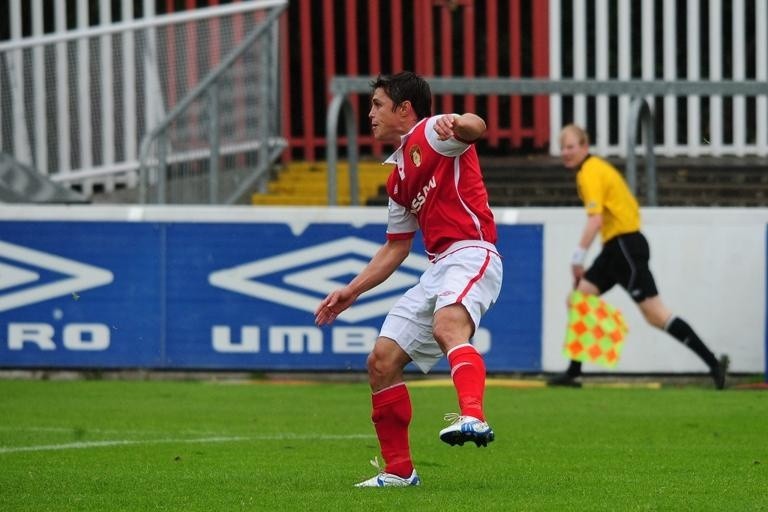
541, 122, 731, 392
311, 70, 506, 489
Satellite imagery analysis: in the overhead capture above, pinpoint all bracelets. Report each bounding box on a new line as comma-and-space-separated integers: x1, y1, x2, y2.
570, 246, 588, 268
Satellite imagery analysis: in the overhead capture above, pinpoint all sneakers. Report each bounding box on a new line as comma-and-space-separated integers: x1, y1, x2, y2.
545, 372, 584, 388
712, 353, 731, 391
438, 411, 496, 448
350, 467, 423, 490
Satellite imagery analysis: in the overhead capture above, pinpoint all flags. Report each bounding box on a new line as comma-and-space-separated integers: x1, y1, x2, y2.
561, 288, 629, 371
